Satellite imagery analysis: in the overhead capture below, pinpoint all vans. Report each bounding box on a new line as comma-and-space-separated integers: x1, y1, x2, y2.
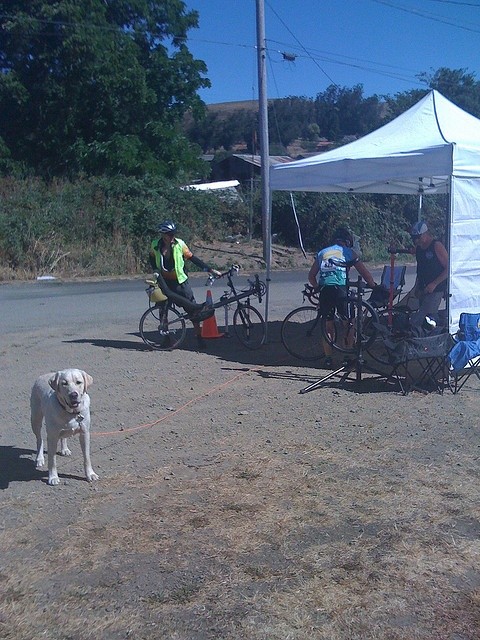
178, 180, 251, 242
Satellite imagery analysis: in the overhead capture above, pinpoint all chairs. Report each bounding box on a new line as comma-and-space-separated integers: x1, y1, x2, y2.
447, 313, 480, 394
365, 292, 448, 395
362, 266, 405, 317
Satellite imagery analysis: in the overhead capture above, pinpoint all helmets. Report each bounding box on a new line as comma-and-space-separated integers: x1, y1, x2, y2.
158, 220, 178, 236
331, 228, 353, 248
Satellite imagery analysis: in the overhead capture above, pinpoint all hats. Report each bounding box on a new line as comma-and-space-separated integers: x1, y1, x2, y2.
410, 221, 428, 236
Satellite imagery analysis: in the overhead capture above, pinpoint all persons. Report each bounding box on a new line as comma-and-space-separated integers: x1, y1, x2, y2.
309, 229, 375, 368
149, 222, 221, 347
412, 222, 449, 327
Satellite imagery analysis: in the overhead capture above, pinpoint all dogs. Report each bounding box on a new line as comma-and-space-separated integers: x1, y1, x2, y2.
31, 369, 100, 486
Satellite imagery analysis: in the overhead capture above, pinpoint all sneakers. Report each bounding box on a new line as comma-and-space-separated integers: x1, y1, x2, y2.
341, 357, 353, 368
320, 356, 335, 369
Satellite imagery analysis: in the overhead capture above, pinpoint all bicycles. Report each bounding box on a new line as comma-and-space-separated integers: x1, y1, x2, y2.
139, 265, 266, 351
281, 275, 419, 361
